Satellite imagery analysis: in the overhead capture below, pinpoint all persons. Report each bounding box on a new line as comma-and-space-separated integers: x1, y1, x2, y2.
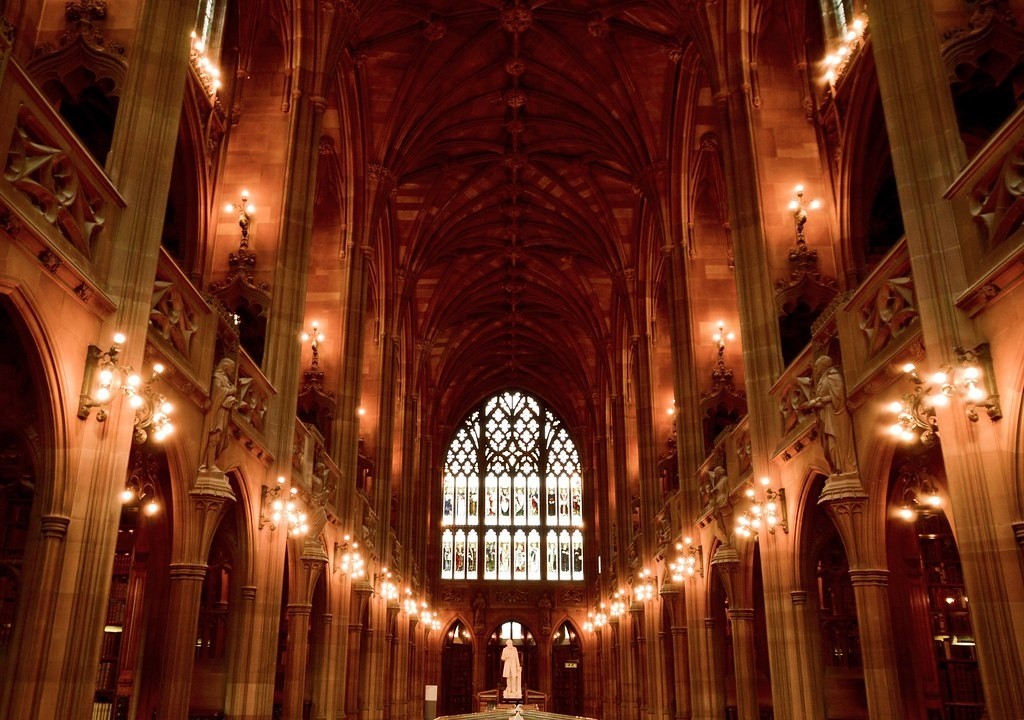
474, 592, 485, 626
200, 358, 239, 472
501, 640, 521, 693
628, 551, 639, 572
312, 463, 327, 500
363, 517, 373, 548
801, 356, 853, 475
538, 593, 551, 628
706, 466, 732, 546
658, 519, 675, 582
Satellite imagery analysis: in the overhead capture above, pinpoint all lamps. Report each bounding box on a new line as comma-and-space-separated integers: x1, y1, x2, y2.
77, 332, 145, 423
224, 188, 256, 253
132, 364, 176, 443
711, 321, 735, 370
581, 479, 784, 631
302, 320, 324, 370
121, 450, 441, 632
881, 363, 939, 448
786, 184, 819, 243
932, 341, 1003, 423
